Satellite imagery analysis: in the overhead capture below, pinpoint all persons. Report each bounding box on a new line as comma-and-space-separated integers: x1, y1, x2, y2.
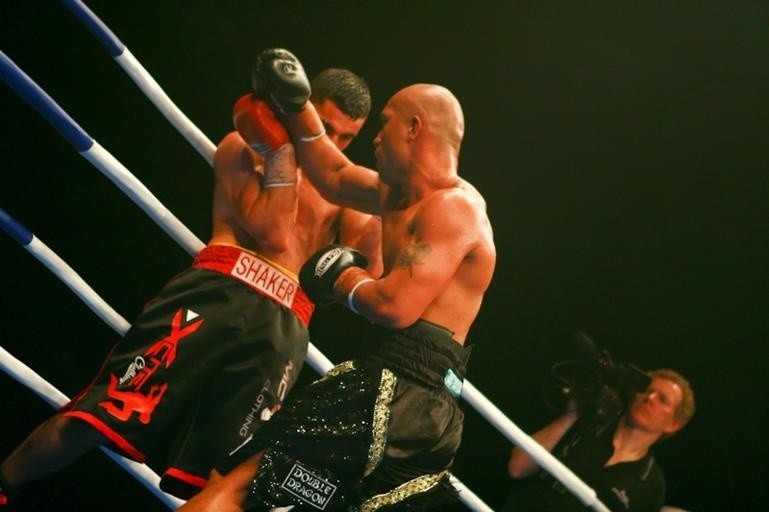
0, 63, 373, 511
506, 365, 699, 511
170, 42, 498, 510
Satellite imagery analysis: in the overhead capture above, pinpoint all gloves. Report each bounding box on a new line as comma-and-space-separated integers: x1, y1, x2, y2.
230, 46, 312, 160
296, 243, 370, 311
